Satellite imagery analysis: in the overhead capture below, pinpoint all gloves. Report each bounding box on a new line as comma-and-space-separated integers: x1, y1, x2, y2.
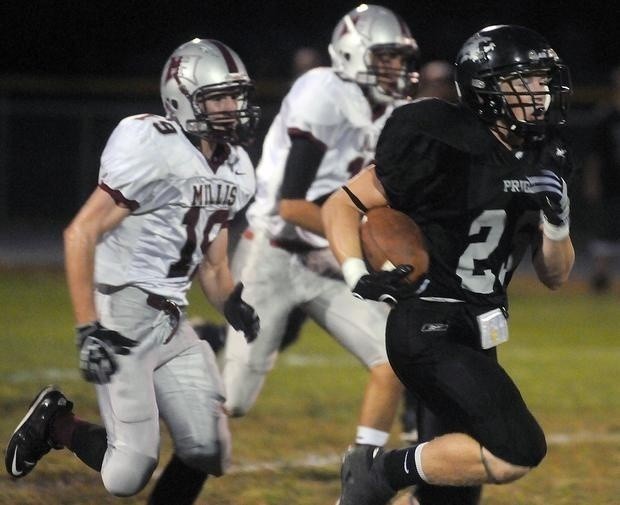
72, 316, 138, 386
342, 256, 431, 307
225, 279, 262, 348
521, 165, 573, 243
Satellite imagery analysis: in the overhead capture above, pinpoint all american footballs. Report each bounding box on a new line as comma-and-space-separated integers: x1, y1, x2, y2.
359, 207, 431, 285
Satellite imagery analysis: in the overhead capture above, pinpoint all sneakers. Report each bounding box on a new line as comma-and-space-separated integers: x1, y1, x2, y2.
2, 380, 76, 479
339, 438, 398, 505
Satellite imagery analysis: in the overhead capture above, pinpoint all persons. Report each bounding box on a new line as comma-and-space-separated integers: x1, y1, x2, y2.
189, 4, 422, 447
5, 37, 261, 505
320, 24, 576, 505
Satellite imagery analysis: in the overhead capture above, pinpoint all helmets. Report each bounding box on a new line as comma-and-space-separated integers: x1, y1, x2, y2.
324, 2, 424, 106
452, 20, 573, 142
160, 35, 262, 147
